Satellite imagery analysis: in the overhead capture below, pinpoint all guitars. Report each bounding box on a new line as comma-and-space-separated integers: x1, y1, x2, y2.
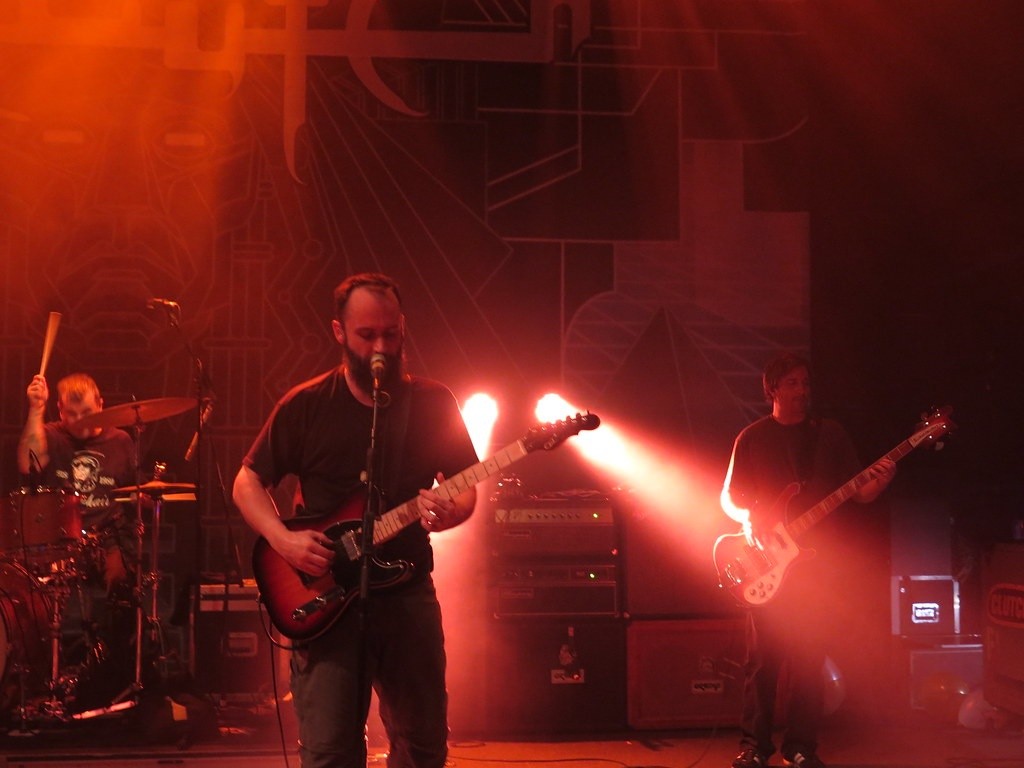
253, 406, 600, 641
712, 403, 959, 606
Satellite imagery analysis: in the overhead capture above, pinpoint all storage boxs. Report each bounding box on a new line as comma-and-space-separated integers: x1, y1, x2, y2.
623, 508, 773, 729
188, 582, 295, 707
887, 571, 983, 717
438, 617, 629, 736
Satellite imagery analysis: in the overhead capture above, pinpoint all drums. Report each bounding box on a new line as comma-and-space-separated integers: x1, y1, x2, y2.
1, 482, 84, 567
0, 557, 52, 721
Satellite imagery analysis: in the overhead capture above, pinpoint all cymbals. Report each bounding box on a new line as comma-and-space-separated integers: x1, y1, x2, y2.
111, 481, 196, 493
71, 395, 198, 427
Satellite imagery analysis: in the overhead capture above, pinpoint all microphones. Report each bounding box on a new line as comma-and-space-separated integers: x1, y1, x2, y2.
147, 298, 179, 310
185, 402, 214, 462
28, 453, 40, 488
370, 353, 385, 388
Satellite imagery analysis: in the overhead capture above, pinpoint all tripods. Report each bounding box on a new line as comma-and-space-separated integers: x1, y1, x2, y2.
103, 421, 171, 714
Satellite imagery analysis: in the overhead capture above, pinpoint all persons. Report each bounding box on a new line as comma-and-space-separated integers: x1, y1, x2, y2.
719, 354, 901, 767
232, 271, 483, 768
16, 372, 176, 710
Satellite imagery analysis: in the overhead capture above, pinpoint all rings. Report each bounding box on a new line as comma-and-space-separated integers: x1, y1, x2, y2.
883, 471, 889, 476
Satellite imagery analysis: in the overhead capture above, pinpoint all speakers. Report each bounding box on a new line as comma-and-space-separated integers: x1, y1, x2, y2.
627, 620, 748, 730
494, 611, 633, 734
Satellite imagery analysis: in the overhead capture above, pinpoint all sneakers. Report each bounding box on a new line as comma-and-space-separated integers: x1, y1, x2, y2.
782, 751, 825, 768
731, 748, 767, 768
